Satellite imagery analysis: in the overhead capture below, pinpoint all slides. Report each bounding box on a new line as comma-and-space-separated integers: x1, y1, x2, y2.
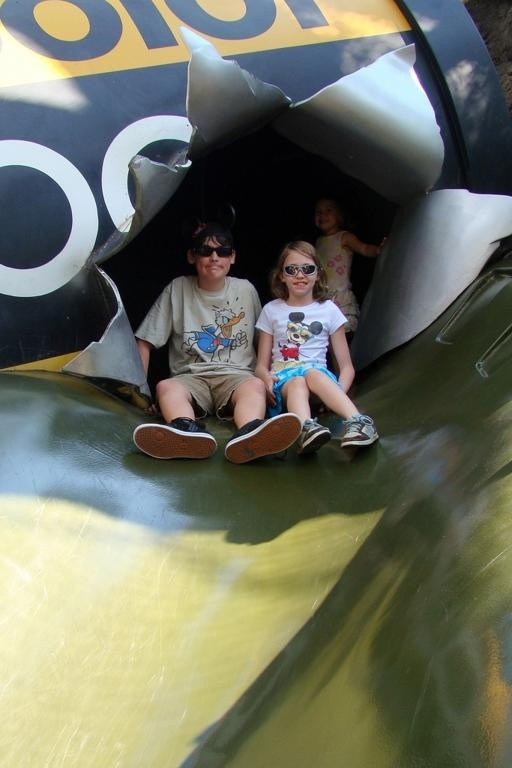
0, 239, 512, 768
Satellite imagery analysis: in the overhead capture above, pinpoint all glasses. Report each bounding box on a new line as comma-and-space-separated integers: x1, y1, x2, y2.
284, 265, 319, 277
195, 245, 233, 257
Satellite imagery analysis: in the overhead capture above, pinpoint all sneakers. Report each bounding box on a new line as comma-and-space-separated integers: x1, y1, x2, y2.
296, 419, 331, 456
341, 415, 379, 448
133, 418, 217, 459
225, 413, 302, 464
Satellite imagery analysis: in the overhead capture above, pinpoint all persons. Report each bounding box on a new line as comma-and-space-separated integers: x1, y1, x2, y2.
312, 198, 387, 375
254, 241, 380, 456
132, 226, 303, 465
